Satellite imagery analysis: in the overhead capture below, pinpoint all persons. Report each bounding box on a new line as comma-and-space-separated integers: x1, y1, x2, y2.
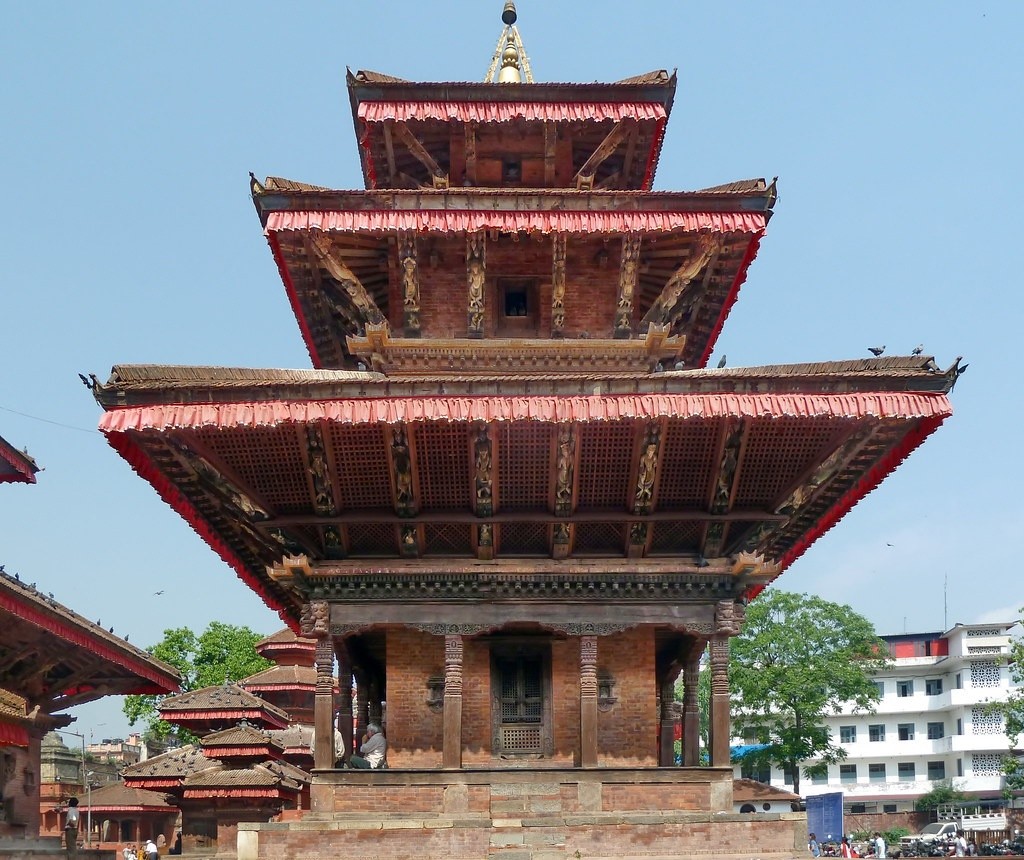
169, 832, 181, 855
122, 840, 158, 860
346, 723, 386, 769
310, 726, 345, 768
65, 797, 79, 860
874, 832, 885, 858
840, 837, 852, 858
947, 830, 978, 857
96, 844, 99, 850
306, 228, 719, 336
154, 422, 873, 549
810, 833, 820, 857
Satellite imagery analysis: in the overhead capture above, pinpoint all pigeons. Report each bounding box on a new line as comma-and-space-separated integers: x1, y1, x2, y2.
717, 355, 726, 368
675, 361, 685, 371
912, 343, 924, 355
655, 363, 664, 371
868, 345, 887, 357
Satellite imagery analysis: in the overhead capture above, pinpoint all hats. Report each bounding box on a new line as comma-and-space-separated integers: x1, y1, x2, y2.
873, 832, 881, 835
146, 840, 151, 843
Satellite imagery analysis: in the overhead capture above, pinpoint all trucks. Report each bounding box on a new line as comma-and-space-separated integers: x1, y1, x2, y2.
897, 798, 1008, 857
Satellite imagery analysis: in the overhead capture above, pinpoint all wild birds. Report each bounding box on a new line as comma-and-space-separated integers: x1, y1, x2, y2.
153, 590, 164, 595
886, 543, 894, 546
119, 679, 302, 777
0, 564, 153, 659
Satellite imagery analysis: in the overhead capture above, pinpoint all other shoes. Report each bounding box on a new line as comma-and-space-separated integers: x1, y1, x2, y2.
334, 761, 345, 768
